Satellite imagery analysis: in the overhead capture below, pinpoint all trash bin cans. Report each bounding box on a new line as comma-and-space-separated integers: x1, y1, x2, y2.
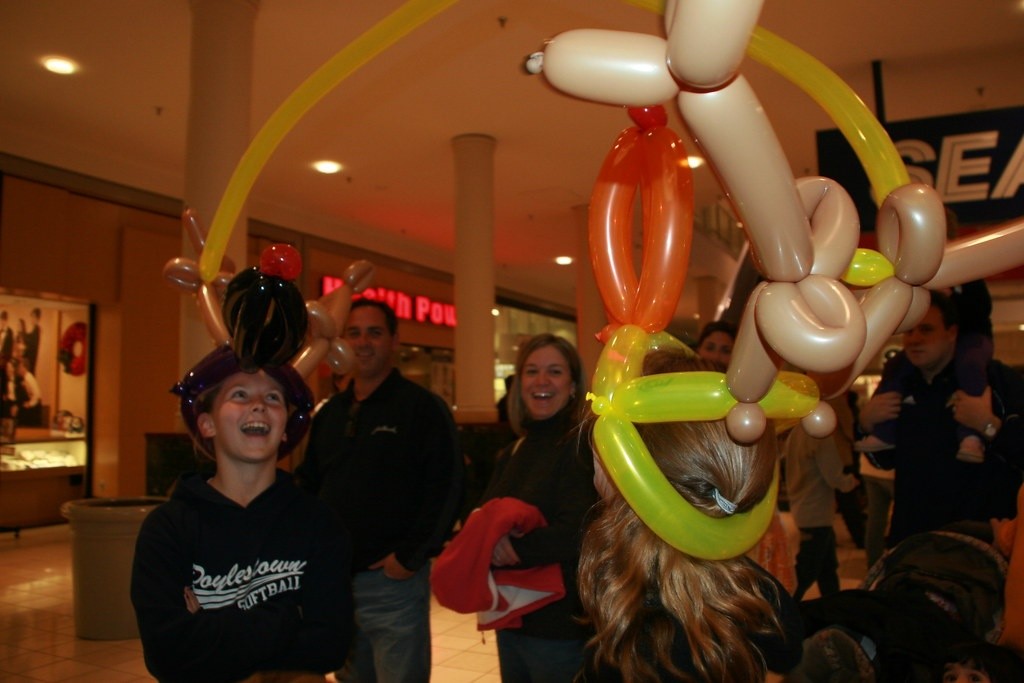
60, 496, 170, 642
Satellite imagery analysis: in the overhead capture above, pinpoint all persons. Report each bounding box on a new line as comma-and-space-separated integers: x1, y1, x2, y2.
296, 298, 483, 682
492, 375, 511, 423
787, 280, 1024, 681
0, 308, 41, 427
473, 334, 603, 683
132, 358, 351, 683
572, 353, 789, 683
695, 322, 735, 372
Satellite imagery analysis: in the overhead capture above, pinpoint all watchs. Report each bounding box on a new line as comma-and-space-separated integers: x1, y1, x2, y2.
981, 417, 1001, 442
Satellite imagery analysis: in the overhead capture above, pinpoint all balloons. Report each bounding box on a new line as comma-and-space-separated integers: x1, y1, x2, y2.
156, 0, 1024, 561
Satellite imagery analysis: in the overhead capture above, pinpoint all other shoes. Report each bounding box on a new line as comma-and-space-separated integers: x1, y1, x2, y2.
956, 434, 985, 463
855, 434, 894, 451
